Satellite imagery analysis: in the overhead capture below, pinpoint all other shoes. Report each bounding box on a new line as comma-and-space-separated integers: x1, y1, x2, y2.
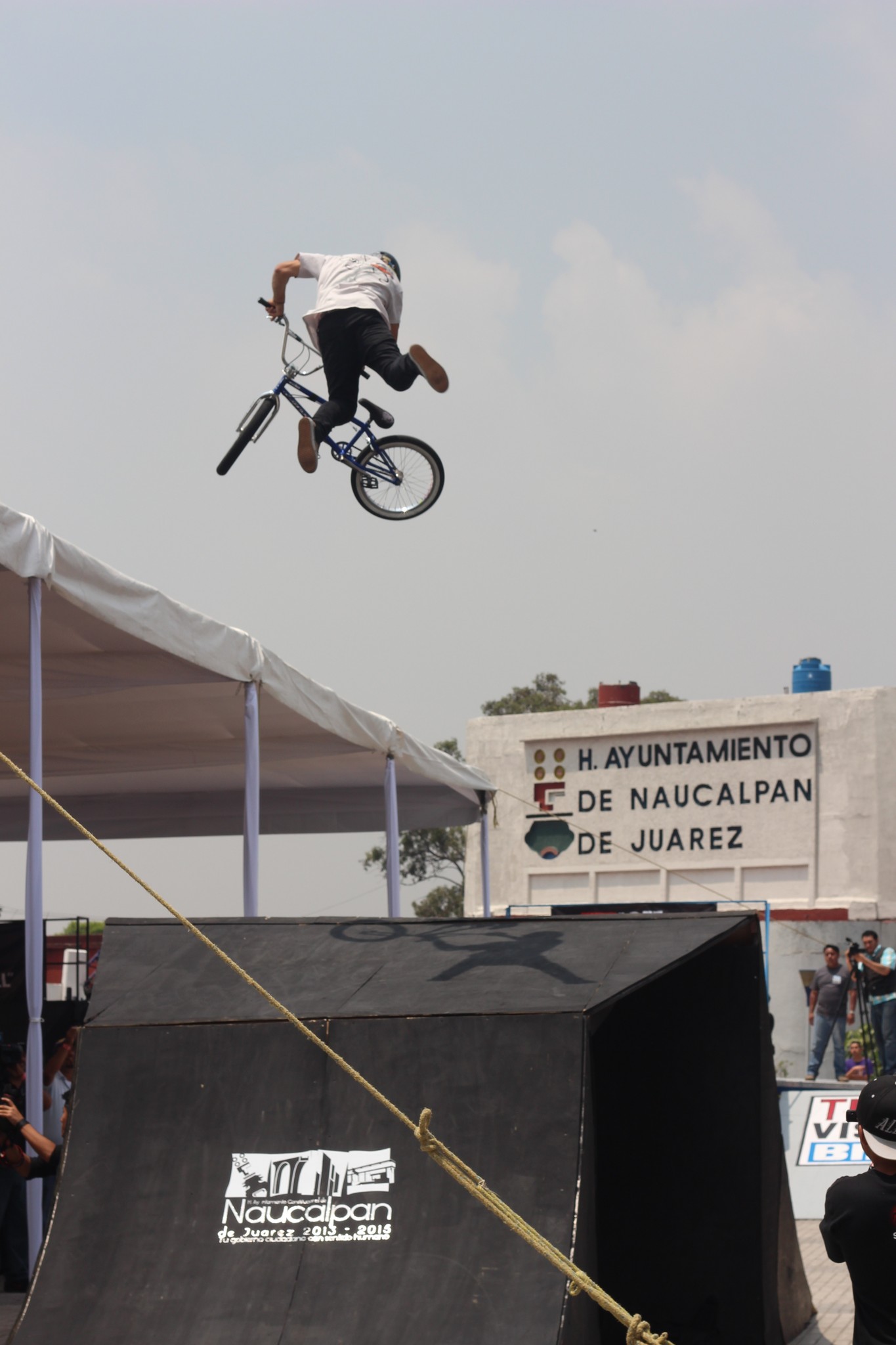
837, 1076, 849, 1082
806, 1074, 815, 1080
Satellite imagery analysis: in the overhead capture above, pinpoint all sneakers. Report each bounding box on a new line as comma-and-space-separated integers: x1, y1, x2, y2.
409, 344, 448, 393
298, 416, 320, 474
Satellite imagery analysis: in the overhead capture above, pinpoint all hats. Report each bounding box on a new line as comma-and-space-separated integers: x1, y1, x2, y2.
855, 1075, 896, 1161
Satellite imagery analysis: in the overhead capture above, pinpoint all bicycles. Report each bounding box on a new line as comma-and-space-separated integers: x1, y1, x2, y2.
215, 297, 446, 521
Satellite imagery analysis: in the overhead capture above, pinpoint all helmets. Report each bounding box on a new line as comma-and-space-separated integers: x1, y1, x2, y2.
376, 251, 401, 282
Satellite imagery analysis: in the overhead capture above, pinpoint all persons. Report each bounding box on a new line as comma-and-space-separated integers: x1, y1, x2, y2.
845, 1040, 873, 1079
820, 1076, 896, 1344
0, 1089, 73, 1243
845, 930, 896, 1075
267, 250, 449, 474
805, 945, 857, 1082
44, 1027, 79, 1145
0, 1043, 52, 1290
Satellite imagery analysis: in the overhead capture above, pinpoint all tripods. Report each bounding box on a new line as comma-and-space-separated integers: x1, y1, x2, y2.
813, 960, 878, 1081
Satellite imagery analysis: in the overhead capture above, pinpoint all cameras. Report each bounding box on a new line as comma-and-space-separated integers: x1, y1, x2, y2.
850, 943, 859, 955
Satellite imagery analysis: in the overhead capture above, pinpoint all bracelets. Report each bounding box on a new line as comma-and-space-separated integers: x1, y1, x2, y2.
5, 1143, 25, 1168
849, 1010, 856, 1015
13, 1118, 29, 1130
